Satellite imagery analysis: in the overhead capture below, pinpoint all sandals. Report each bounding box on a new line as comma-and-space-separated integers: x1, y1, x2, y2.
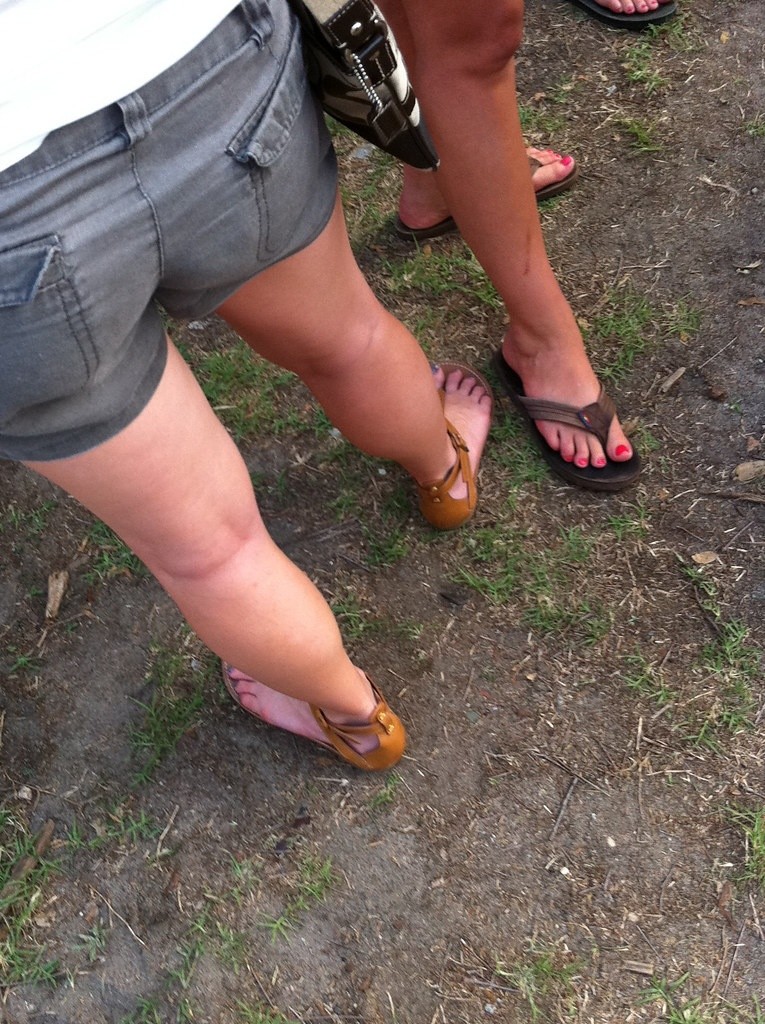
220, 658, 406, 772
414, 359, 493, 531
570, 0, 678, 30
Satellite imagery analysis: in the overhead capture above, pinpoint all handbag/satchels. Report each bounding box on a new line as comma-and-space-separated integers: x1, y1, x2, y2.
293, 0, 441, 173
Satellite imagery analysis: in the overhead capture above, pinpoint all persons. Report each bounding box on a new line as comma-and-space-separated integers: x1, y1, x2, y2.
0, 1, 495, 771
377, 1, 646, 494
575, 0, 678, 30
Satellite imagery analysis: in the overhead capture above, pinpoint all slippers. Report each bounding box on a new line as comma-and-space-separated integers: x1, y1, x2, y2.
494, 336, 642, 492
395, 144, 579, 242
